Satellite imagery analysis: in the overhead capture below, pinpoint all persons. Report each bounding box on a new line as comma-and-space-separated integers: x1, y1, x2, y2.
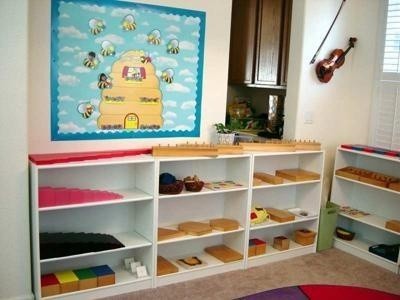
83, 51, 96, 67
98, 73, 110, 88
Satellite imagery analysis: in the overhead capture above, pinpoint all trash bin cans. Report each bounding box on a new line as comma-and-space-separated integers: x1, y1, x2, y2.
317, 202, 340, 251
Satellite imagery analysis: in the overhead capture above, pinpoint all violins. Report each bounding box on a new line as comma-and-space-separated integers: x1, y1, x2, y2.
316, 37, 358, 83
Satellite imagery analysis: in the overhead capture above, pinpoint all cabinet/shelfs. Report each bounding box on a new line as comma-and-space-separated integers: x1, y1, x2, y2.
229, 0, 293, 90
28, 154, 156, 300
153, 153, 252, 289
245, 150, 324, 271
330, 146, 400, 274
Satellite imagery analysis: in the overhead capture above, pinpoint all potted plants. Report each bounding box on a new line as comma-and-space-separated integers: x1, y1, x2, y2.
214, 118, 242, 145
